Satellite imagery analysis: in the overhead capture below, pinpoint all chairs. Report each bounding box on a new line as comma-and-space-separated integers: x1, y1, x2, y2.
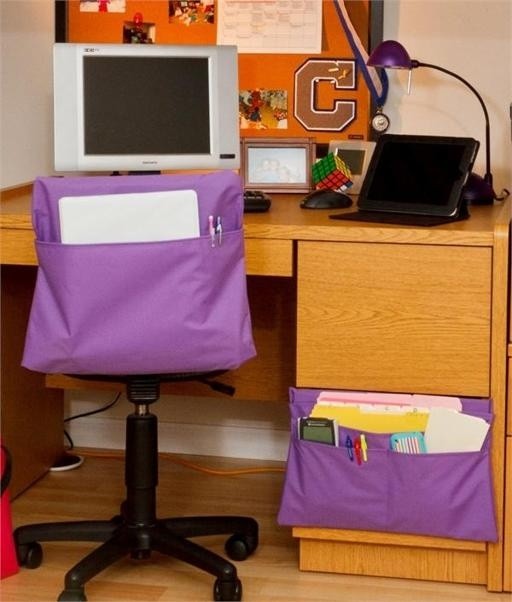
9, 168, 262, 602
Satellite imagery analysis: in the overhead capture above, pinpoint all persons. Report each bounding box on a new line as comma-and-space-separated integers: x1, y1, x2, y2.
124, 28, 153, 45
169, 0, 214, 22
97, 0, 111, 12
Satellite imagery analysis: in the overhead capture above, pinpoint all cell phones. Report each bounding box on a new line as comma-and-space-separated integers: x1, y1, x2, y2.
297, 416, 339, 448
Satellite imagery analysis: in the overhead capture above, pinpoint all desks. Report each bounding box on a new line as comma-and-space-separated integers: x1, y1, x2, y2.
3, 172, 512, 594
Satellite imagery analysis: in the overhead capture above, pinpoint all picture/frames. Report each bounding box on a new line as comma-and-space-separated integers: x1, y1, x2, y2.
241, 136, 318, 197
326, 138, 376, 197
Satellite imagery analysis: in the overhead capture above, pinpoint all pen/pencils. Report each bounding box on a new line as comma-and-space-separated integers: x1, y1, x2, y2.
208, 216, 222, 247
346, 433, 368, 465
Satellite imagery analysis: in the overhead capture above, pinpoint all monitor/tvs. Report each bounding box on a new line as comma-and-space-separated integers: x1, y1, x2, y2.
53, 43, 239, 172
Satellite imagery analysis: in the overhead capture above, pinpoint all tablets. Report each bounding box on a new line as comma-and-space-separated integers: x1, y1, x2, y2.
356, 133, 480, 218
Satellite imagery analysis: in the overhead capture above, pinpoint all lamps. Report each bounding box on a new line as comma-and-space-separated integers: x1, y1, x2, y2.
364, 37, 512, 206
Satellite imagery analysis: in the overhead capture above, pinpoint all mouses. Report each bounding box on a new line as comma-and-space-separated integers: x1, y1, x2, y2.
300, 189, 353, 209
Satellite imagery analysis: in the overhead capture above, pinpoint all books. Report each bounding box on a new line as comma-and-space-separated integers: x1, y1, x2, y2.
309, 391, 491, 452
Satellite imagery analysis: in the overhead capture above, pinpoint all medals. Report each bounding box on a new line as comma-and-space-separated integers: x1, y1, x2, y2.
371, 112, 391, 134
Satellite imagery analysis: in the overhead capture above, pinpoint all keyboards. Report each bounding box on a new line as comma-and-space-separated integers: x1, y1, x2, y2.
243, 189, 271, 212
